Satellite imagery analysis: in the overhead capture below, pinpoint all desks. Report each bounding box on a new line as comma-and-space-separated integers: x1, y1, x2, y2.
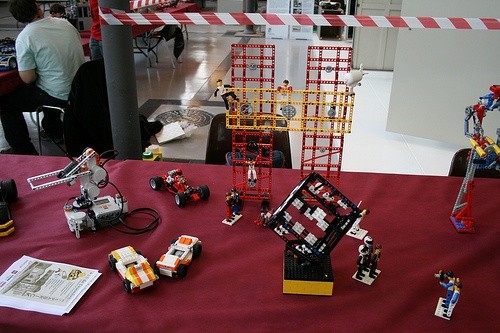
0, 155, 500, 333
0, 3, 202, 96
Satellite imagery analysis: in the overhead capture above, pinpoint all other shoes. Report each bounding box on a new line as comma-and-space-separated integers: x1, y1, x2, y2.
0, 147, 39, 155
40, 130, 48, 137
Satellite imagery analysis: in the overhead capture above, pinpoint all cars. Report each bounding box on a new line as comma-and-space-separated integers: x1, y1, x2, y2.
108, 245, 159, 294
155, 234, 202, 279
150, 169, 209, 207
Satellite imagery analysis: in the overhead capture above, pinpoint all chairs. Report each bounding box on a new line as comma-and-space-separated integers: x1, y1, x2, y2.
207, 112, 292, 170
36, 59, 113, 156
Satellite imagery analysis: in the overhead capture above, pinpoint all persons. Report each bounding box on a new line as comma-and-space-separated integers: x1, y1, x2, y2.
356, 236, 382, 280
246, 157, 257, 187
214, 79, 240, 110
225, 188, 242, 222
277, 80, 292, 110
260, 194, 272, 226
0, 0, 105, 156
441, 273, 463, 319
